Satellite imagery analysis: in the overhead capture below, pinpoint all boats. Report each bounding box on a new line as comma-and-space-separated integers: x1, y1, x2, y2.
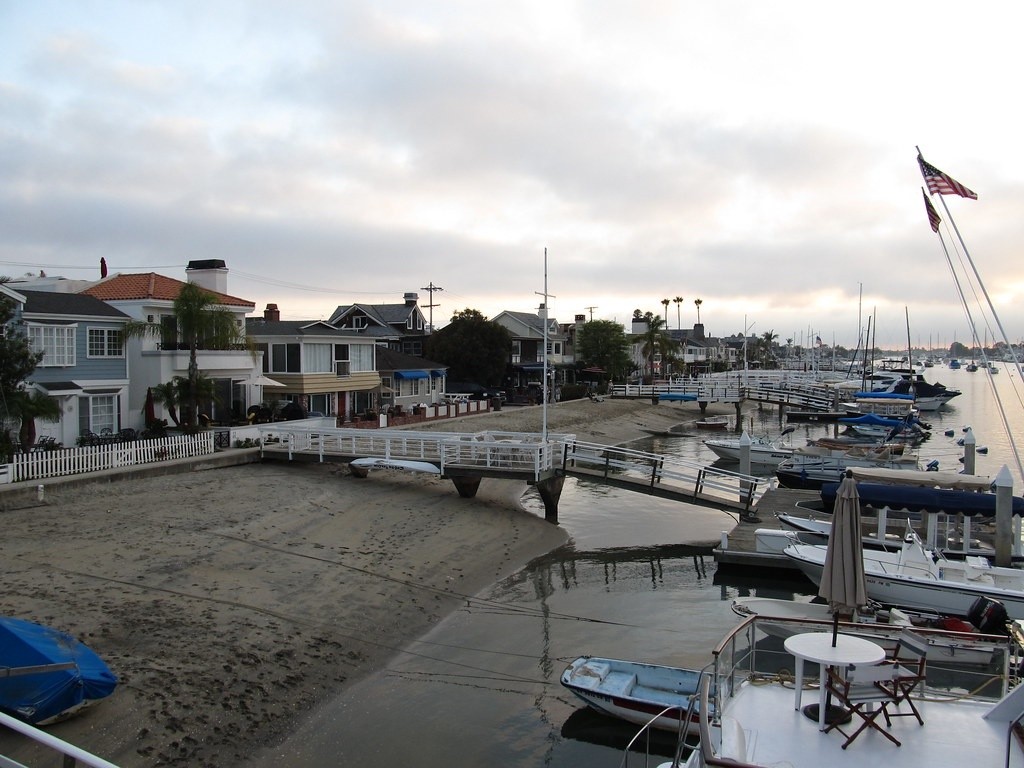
784, 515, 1024, 627
735, 596, 1024, 674
560, 654, 725, 735
616, 616, 1024, 768
772, 508, 1024, 565
663, 282, 1024, 541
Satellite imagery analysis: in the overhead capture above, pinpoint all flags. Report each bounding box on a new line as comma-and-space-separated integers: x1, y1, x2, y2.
921, 188, 943, 232
916, 156, 978, 199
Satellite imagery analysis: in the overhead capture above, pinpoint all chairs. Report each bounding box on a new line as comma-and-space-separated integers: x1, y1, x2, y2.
825, 668, 900, 750
12, 405, 274, 456
869, 626, 930, 727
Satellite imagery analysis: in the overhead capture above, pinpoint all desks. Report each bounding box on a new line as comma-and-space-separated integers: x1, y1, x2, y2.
783, 633, 885, 732
